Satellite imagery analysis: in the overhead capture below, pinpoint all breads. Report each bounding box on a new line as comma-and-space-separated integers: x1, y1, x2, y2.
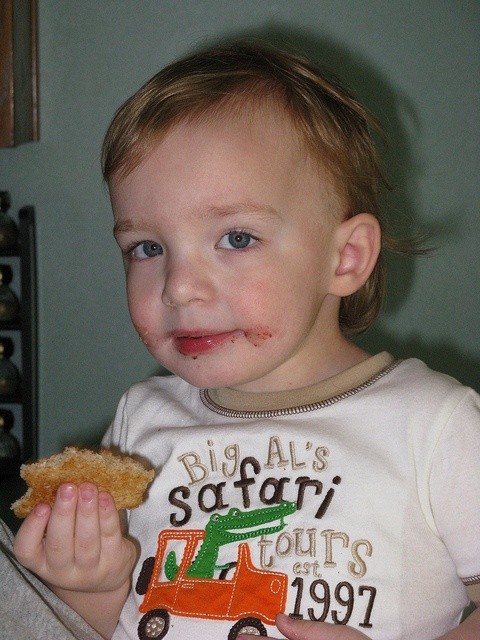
9, 446, 157, 519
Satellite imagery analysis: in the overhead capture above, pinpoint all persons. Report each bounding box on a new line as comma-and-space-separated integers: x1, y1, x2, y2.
13, 43, 480, 640
